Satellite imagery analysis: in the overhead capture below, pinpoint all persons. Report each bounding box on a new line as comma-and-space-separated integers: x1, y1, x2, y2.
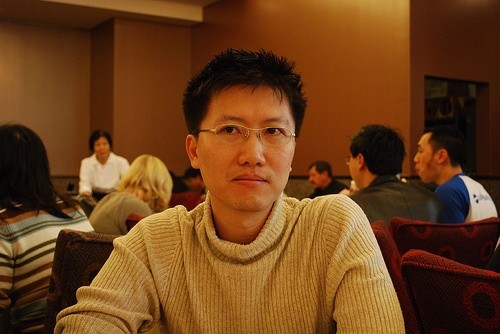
79, 129, 208, 235
54, 48, 406, 334
0, 122, 95, 334
305, 123, 497, 224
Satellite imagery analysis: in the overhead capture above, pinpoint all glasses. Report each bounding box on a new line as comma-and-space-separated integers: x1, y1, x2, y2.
346, 156, 363, 165
188, 123, 297, 147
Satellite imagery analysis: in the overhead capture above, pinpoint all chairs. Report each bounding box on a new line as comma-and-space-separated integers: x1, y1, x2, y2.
43, 229, 122, 334
370, 216, 500, 334
